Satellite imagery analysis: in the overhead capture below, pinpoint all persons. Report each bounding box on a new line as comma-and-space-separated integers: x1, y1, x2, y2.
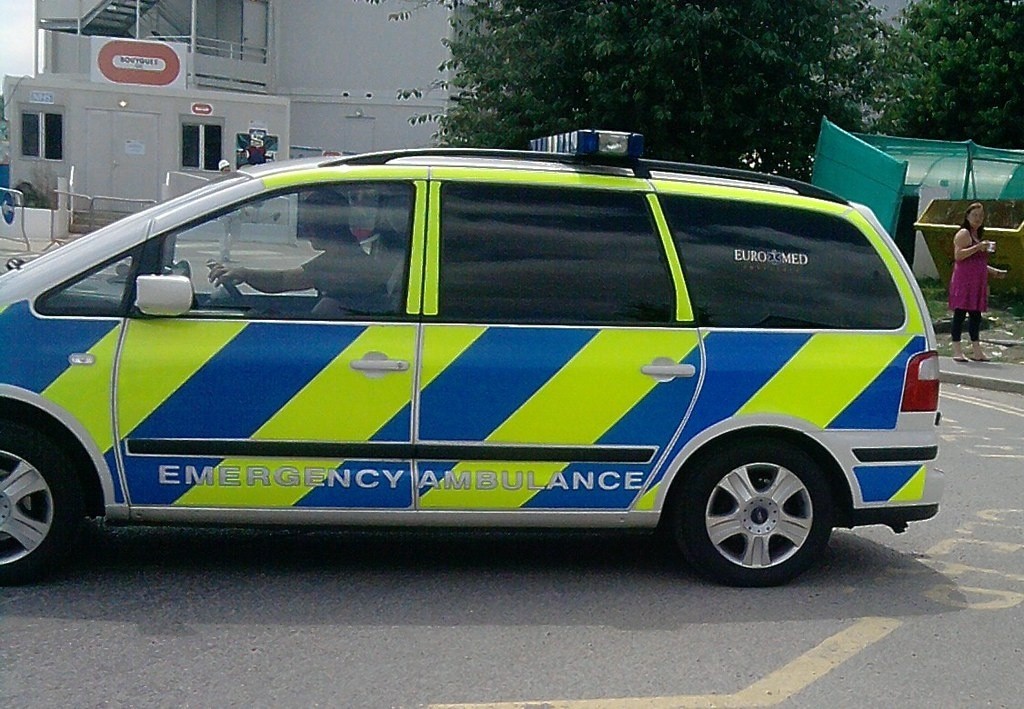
948, 202, 1008, 363
205, 189, 390, 316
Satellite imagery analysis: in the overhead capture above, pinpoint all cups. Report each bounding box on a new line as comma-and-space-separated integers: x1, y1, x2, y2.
987, 241, 996, 252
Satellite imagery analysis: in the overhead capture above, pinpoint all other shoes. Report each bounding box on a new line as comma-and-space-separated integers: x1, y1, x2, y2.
971, 355, 990, 361
952, 355, 968, 362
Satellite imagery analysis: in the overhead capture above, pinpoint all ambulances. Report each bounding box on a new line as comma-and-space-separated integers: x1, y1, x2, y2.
1, 127, 940, 589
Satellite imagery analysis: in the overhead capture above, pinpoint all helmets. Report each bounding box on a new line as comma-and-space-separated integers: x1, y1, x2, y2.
219, 160, 230, 170
